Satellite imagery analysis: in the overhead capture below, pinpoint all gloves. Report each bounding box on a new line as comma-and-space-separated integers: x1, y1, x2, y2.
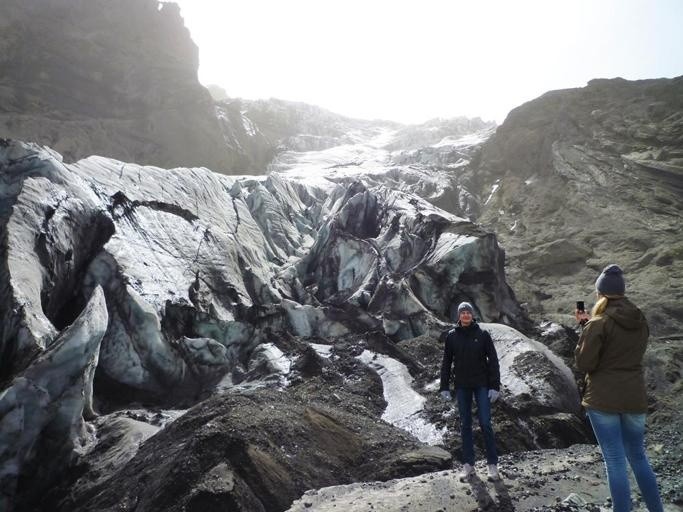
440, 390, 453, 401
487, 389, 499, 403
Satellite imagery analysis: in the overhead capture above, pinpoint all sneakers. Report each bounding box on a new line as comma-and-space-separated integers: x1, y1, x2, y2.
458, 463, 476, 482
486, 464, 500, 481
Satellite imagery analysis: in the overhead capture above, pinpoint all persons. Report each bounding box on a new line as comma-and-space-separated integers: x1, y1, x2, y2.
440, 301, 500, 480
572, 263, 663, 512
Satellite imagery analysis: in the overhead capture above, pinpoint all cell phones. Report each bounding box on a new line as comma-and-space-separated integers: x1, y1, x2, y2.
577, 301, 584, 313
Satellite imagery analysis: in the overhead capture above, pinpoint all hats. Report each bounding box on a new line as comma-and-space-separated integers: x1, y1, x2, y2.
594, 264, 625, 295
457, 301, 473, 317
